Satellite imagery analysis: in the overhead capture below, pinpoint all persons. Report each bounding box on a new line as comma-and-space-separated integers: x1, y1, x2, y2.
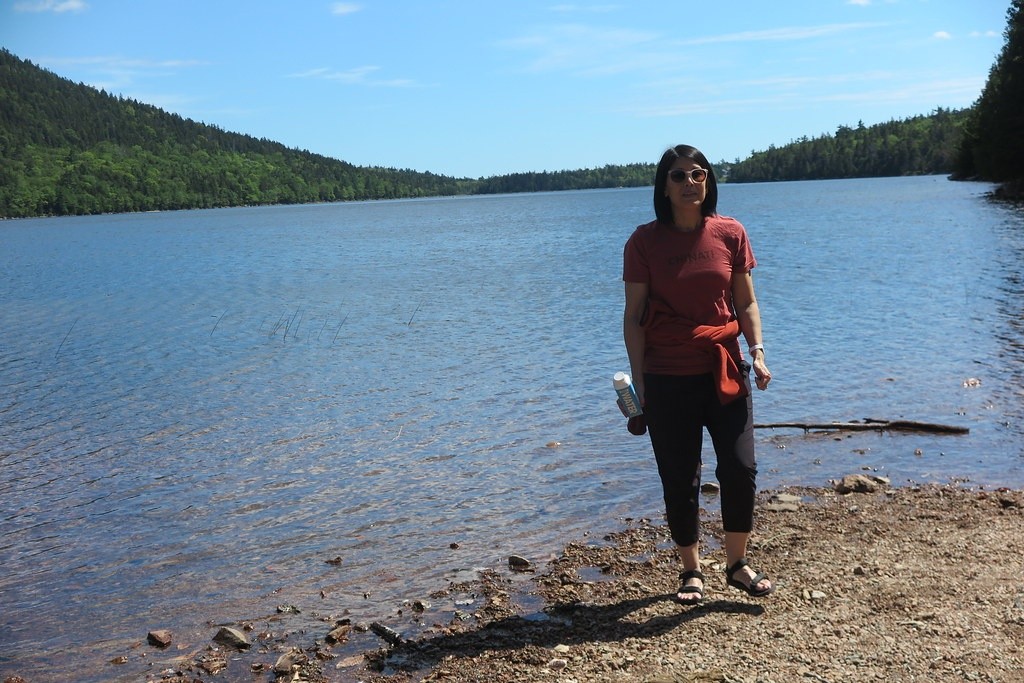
616, 145, 772, 604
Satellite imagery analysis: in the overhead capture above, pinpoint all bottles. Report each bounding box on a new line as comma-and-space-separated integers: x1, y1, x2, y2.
612, 371, 643, 418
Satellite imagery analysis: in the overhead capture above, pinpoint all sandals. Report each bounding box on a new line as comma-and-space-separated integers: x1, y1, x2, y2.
676, 570, 705, 605
726, 558, 776, 597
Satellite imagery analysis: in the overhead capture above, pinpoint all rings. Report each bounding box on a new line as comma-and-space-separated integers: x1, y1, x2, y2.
766, 387, 768, 389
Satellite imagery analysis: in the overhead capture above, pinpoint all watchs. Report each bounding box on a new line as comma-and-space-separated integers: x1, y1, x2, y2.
749, 344, 765, 357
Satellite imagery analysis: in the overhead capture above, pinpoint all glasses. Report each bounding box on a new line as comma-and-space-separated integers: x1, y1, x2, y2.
667, 169, 708, 184
